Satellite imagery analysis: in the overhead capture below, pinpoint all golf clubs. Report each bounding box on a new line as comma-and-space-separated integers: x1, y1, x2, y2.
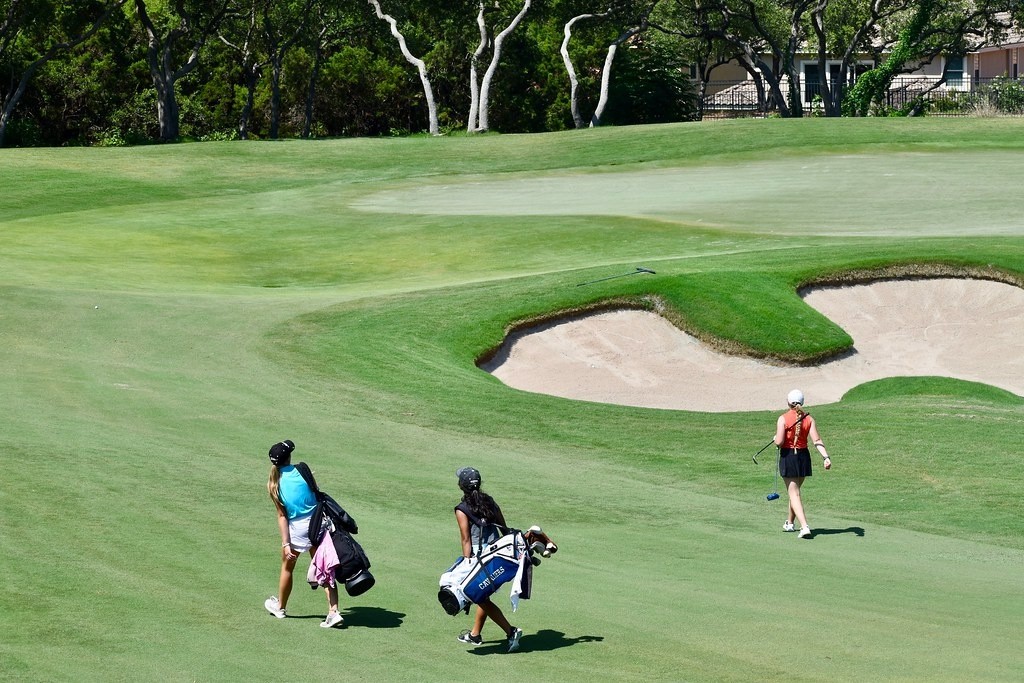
521, 525, 559, 567
752, 411, 810, 466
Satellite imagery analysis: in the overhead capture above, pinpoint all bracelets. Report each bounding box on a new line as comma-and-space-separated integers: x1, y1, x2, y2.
823, 456, 830, 460
283, 542, 290, 548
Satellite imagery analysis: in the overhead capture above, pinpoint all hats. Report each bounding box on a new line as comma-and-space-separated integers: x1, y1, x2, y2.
456, 466, 481, 490
269, 440, 295, 465
788, 389, 804, 407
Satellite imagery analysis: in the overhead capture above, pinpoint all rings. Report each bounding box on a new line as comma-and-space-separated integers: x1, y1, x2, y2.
288, 555, 290, 557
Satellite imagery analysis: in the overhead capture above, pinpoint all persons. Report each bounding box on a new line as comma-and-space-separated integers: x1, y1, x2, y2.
453, 466, 522, 654
263, 440, 345, 628
774, 389, 832, 539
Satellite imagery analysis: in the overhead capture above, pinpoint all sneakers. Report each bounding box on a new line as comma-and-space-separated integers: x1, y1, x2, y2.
457, 629, 482, 645
265, 596, 286, 619
320, 611, 344, 628
506, 626, 522, 652
798, 525, 810, 538
782, 520, 794, 532
